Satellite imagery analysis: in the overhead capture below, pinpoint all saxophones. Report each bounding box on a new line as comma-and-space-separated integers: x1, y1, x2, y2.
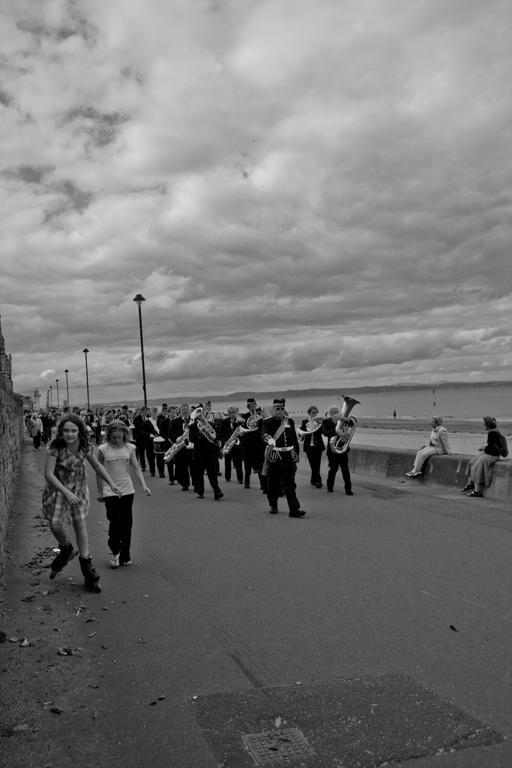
221, 425, 242, 454
164, 429, 189, 463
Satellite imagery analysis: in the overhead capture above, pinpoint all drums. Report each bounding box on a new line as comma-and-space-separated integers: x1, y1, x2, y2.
153, 435, 166, 455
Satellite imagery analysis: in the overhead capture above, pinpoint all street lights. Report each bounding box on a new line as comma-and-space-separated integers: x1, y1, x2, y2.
132, 294, 150, 410
82, 348, 93, 413
64, 369, 70, 409
55, 379, 60, 410
46, 385, 53, 409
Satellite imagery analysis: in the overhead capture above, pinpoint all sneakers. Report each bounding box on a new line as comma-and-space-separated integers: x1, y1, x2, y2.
215, 472, 250, 489
215, 492, 223, 500
461, 483, 474, 492
405, 471, 422, 477
345, 490, 354, 495
150, 473, 155, 477
182, 487, 205, 499
270, 507, 278, 514
160, 474, 165, 478
289, 510, 306, 517
109, 552, 120, 568
466, 491, 483, 497
312, 483, 323, 488
122, 560, 132, 565
328, 487, 334, 492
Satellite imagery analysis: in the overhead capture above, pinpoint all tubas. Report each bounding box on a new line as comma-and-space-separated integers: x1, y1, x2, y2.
330, 394, 361, 454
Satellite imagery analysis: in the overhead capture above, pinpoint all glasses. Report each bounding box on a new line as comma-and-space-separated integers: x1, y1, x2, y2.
276, 408, 285, 411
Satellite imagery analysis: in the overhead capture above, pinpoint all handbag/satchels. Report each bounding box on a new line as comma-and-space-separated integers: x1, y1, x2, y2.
487, 430, 509, 457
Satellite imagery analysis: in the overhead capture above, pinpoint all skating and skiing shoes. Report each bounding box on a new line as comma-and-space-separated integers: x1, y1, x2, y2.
80, 553, 102, 593
49, 537, 79, 579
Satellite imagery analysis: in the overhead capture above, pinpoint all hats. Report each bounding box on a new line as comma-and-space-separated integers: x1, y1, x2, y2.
273, 397, 286, 406
483, 415, 497, 427
432, 415, 442, 425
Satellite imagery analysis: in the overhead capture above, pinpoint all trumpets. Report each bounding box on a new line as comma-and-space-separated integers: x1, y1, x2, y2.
190, 407, 216, 443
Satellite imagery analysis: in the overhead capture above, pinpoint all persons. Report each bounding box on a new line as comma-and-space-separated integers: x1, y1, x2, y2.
298, 405, 324, 488
41, 413, 123, 594
460, 416, 501, 498
22, 396, 306, 517
322, 405, 357, 495
405, 415, 453, 477
97, 418, 149, 569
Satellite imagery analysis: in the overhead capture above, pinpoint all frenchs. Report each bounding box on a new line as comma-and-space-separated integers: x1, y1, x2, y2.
239, 412, 262, 436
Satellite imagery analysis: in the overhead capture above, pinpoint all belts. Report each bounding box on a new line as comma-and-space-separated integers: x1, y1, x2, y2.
266, 446, 294, 452
